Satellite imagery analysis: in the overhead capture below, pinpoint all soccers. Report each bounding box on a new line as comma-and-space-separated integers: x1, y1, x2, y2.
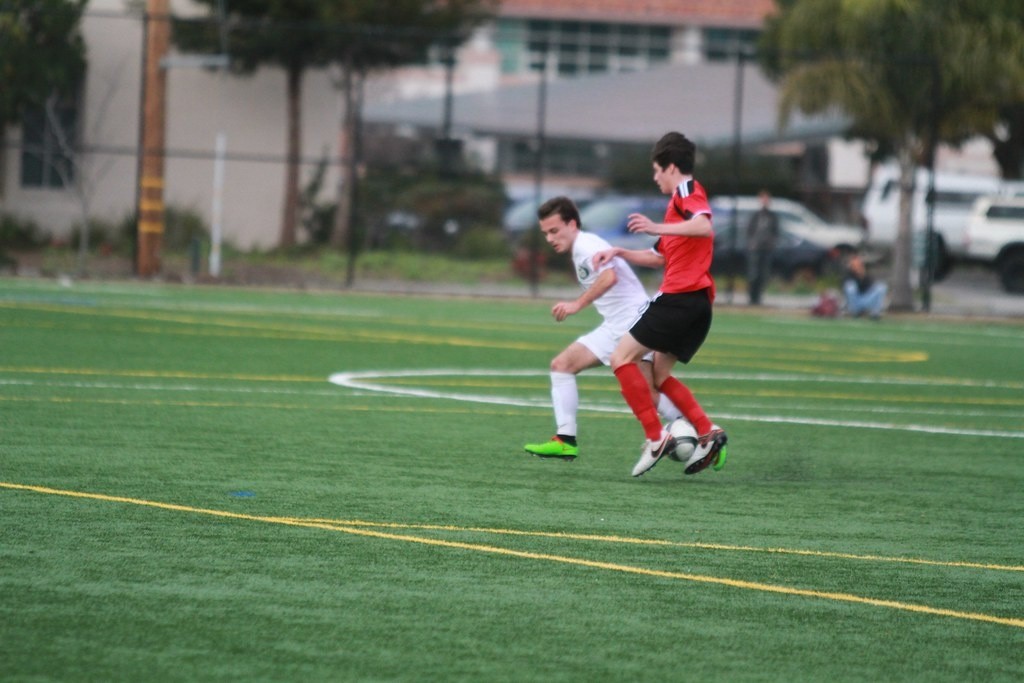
662, 414, 699, 462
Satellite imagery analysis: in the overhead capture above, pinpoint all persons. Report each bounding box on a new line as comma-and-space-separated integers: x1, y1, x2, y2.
523, 196, 727, 472
744, 189, 780, 304
592, 132, 728, 476
840, 259, 887, 319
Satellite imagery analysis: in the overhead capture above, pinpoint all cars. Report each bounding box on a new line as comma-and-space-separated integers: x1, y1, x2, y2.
503, 165, 1024, 296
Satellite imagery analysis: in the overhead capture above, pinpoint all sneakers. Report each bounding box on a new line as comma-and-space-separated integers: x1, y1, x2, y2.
684, 424, 728, 474
523, 436, 578, 462
632, 429, 676, 476
712, 444, 726, 470
